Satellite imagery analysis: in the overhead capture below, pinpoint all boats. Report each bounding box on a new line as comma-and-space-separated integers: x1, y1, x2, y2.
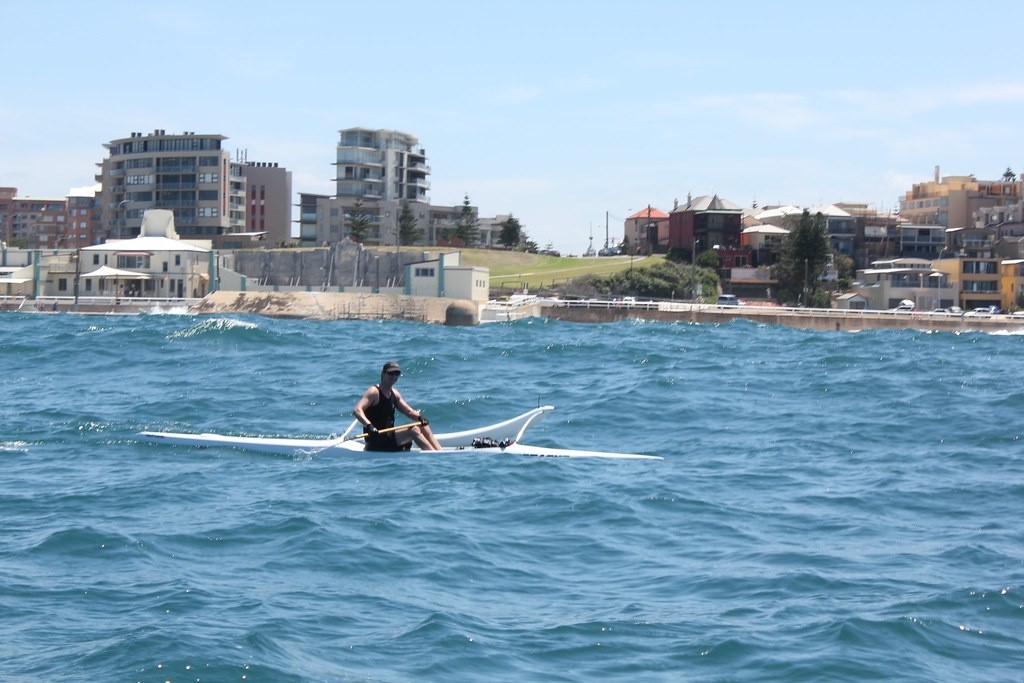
132, 391, 667, 461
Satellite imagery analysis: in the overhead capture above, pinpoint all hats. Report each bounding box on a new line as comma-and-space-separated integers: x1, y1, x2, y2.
383, 361, 403, 375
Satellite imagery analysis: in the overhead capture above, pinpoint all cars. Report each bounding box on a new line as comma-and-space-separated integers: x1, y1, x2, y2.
888, 304, 1024, 319
488, 290, 636, 307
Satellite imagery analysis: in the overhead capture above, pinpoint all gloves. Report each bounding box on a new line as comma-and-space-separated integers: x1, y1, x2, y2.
366, 424, 378, 436
418, 416, 429, 426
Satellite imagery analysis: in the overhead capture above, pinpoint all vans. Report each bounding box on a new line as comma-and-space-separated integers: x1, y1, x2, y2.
717, 294, 738, 310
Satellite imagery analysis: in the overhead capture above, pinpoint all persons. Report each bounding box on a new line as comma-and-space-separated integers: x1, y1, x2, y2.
353, 360, 443, 453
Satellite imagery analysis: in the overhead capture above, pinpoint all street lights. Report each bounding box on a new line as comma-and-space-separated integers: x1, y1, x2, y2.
692, 237, 699, 303
938, 246, 948, 308
71, 248, 80, 303
117, 199, 130, 240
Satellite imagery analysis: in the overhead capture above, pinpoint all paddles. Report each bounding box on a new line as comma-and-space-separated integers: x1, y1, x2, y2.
301, 420, 424, 455
